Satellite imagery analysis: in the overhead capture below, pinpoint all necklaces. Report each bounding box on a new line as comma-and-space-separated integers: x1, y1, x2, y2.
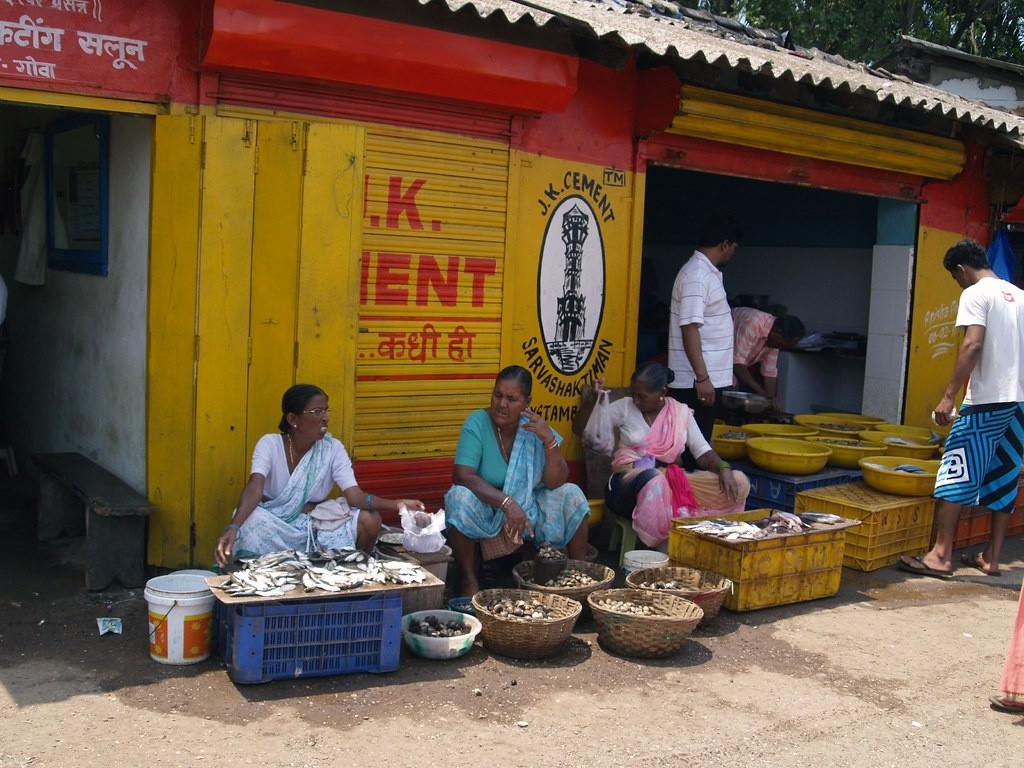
645, 412, 652, 426
497, 427, 509, 462
286, 433, 294, 464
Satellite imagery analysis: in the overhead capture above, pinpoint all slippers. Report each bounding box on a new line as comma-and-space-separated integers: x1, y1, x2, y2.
896, 555, 955, 575
988, 693, 1023, 712
962, 551, 1001, 575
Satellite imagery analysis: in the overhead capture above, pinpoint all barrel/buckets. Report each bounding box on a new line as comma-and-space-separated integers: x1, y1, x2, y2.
623, 551, 670, 587
534, 553, 566, 585
144, 569, 218, 666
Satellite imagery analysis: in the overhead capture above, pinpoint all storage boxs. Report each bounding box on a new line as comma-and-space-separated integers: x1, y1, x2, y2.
668, 457, 1024, 612
212, 591, 403, 684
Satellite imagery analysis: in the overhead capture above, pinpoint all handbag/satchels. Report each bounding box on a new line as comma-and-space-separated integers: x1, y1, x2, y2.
582, 389, 615, 455
396, 505, 447, 553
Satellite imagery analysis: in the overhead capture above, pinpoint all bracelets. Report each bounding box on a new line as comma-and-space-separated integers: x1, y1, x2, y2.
696, 375, 709, 383
715, 460, 733, 471
367, 495, 374, 512
500, 496, 515, 512
543, 436, 558, 452
225, 524, 241, 540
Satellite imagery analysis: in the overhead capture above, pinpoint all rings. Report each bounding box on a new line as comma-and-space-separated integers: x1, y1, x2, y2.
702, 398, 705, 400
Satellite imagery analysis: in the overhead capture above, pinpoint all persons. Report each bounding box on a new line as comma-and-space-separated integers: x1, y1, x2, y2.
896, 240, 1024, 578
570, 362, 750, 550
212, 383, 425, 569
728, 306, 805, 410
444, 363, 591, 599
667, 212, 736, 471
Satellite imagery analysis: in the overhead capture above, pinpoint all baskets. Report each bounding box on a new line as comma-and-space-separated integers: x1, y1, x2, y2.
625, 567, 734, 627
586, 587, 704, 658
448, 597, 476, 616
564, 541, 598, 561
512, 558, 615, 606
471, 586, 583, 658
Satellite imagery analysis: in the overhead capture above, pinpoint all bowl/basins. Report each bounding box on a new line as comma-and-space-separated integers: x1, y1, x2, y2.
709, 413, 949, 496
400, 610, 482, 659
721, 391, 774, 412
448, 597, 476, 617
587, 499, 605, 527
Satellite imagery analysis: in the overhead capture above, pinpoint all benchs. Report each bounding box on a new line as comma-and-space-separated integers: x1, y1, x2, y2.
31, 452, 155, 591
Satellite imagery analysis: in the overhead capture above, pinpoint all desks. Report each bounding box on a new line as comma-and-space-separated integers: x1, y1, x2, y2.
774, 349, 867, 419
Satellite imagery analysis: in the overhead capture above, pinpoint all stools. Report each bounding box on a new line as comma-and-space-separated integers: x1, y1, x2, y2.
608, 514, 638, 569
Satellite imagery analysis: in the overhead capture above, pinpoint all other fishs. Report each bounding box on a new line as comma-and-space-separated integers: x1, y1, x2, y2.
212, 549, 427, 597
676, 510, 845, 539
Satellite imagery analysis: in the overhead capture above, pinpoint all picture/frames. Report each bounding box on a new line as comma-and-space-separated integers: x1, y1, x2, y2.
46, 114, 109, 277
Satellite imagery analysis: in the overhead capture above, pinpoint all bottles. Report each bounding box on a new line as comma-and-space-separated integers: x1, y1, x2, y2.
209, 564, 221, 575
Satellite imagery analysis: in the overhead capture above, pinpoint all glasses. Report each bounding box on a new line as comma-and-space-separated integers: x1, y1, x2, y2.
298, 408, 333, 419
